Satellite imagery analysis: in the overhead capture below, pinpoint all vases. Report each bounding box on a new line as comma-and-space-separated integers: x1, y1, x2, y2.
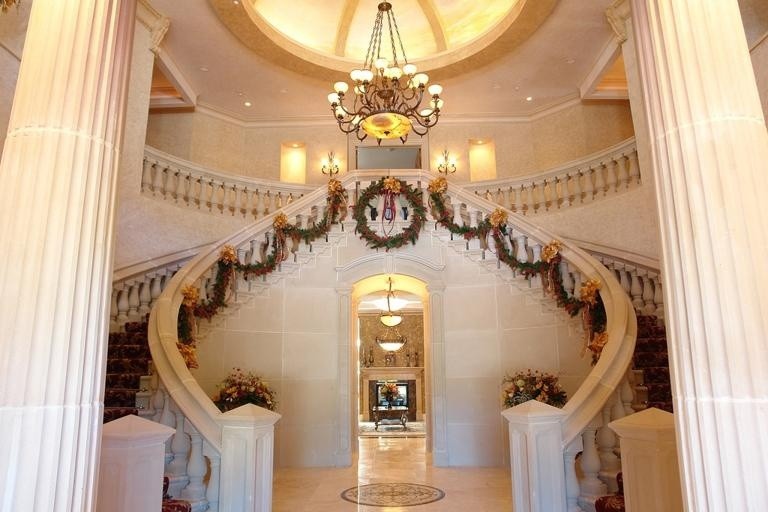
386, 396, 394, 408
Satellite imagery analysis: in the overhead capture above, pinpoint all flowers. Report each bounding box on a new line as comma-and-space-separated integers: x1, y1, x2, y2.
500, 369, 568, 412
350, 179, 427, 251
212, 366, 277, 412
379, 383, 400, 397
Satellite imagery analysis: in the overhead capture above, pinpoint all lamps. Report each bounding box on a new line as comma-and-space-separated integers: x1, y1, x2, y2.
326, 0, 444, 147
322, 151, 339, 177
373, 277, 408, 354
437, 149, 456, 174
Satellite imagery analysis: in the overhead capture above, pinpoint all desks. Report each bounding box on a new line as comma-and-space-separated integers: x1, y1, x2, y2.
373, 406, 408, 431
